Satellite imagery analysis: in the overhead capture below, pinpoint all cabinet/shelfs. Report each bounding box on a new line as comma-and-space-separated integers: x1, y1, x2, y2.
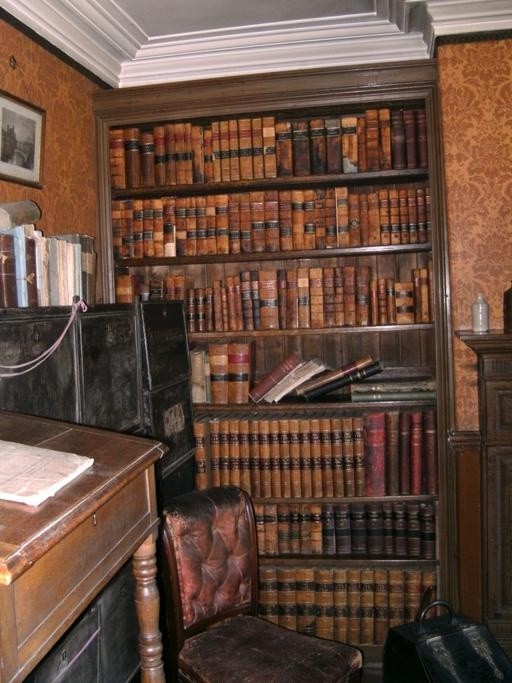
0, 299, 199, 683
84, 58, 454, 662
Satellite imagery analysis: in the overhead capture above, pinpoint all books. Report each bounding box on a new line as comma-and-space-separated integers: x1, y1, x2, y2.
191, 339, 437, 404
0, 223, 99, 310
110, 110, 426, 190
259, 564, 437, 645
116, 266, 429, 333
254, 502, 435, 559
195, 409, 437, 497
1, 439, 97, 508
112, 187, 430, 260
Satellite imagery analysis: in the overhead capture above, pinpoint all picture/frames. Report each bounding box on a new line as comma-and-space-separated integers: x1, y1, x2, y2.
0, 89, 48, 190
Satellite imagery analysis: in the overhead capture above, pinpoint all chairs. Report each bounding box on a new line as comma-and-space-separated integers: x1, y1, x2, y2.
159, 485, 363, 682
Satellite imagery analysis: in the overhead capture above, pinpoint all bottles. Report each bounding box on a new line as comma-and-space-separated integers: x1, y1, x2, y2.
504, 281, 512, 334
471, 292, 489, 333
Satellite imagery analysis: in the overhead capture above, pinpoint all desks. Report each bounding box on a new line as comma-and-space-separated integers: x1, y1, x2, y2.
1, 409, 165, 682
455, 330, 511, 664
